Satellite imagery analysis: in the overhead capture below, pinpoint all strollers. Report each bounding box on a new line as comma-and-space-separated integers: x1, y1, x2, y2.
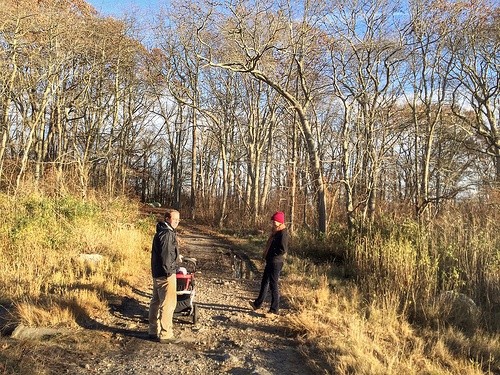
174, 258, 198, 324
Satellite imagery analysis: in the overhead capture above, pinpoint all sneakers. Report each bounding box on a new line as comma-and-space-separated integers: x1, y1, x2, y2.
267, 312, 279, 315
149, 330, 159, 342
159, 335, 182, 344
249, 300, 257, 310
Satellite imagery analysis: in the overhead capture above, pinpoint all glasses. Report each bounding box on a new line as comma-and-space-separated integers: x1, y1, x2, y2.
165, 217, 181, 222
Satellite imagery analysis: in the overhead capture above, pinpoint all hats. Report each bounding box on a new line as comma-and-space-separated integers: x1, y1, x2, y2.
271, 212, 285, 224
176, 267, 187, 275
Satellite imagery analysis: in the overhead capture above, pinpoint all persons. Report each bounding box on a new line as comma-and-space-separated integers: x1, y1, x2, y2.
176, 266, 187, 275
147, 209, 183, 344
248, 211, 288, 314
